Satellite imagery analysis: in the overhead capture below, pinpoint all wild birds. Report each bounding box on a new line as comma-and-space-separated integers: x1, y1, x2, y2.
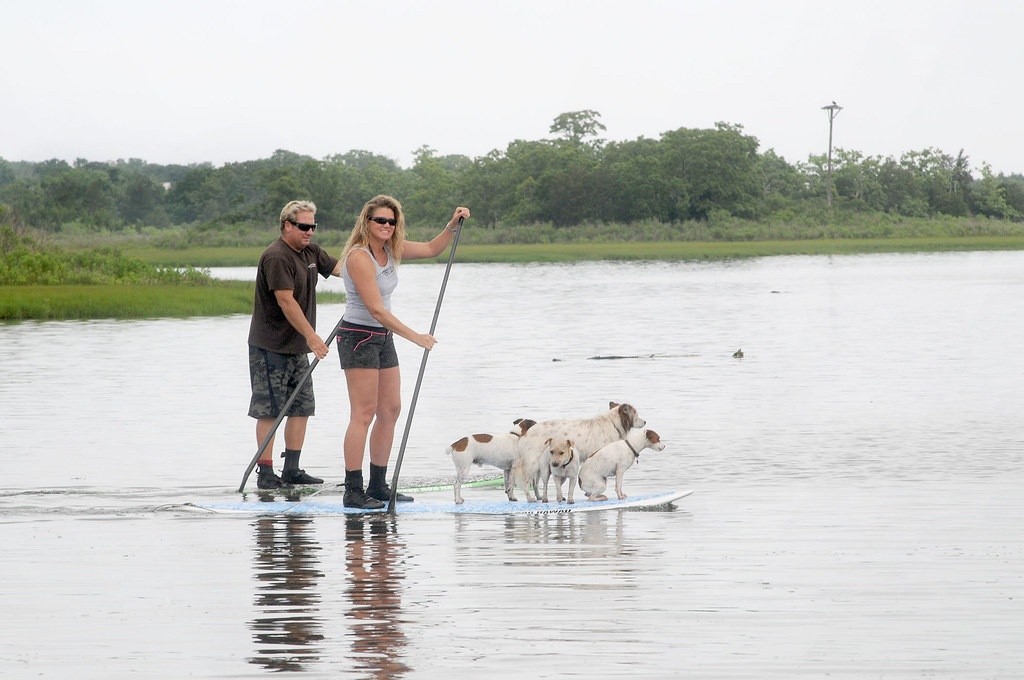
733, 348, 743, 358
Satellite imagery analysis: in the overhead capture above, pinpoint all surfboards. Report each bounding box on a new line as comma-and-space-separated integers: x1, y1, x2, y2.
163, 488, 694, 514
112, 473, 506, 496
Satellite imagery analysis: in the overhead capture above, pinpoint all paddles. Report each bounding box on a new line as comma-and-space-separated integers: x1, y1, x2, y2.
386, 216, 464, 512
237, 314, 345, 494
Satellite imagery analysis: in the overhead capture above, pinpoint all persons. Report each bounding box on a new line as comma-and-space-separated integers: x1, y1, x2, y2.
248, 201, 344, 489
336, 194, 470, 511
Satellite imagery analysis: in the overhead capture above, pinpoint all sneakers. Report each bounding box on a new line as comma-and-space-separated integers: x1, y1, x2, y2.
257, 475, 295, 490
281, 471, 324, 485
342, 483, 386, 509
364, 483, 416, 502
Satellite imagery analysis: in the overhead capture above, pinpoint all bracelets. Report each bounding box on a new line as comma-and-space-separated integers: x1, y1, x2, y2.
446, 221, 459, 232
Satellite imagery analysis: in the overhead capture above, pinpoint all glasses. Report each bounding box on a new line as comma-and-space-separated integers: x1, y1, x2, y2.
288, 219, 318, 232
368, 217, 397, 226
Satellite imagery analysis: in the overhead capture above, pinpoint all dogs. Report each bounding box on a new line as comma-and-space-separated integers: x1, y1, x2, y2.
505, 401, 666, 504
445, 418, 538, 505
